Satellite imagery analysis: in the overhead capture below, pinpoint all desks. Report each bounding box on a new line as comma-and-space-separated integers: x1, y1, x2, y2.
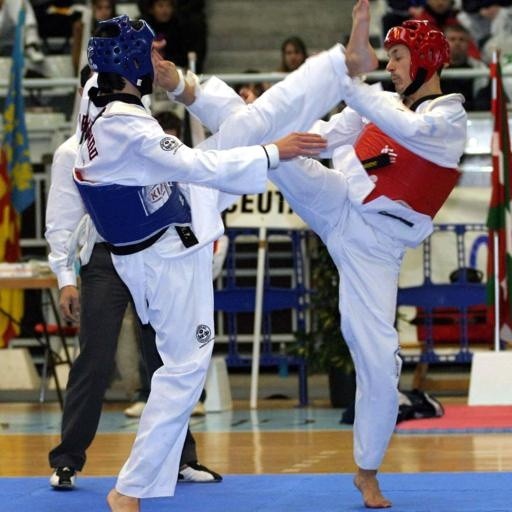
0, 271, 80, 410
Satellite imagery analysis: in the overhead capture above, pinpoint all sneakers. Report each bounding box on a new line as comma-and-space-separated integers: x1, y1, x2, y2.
50, 467, 77, 490
178, 465, 223, 482
122, 402, 147, 418
189, 400, 205, 416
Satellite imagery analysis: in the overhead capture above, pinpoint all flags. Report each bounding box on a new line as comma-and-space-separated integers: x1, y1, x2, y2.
0, 2, 37, 212
0, 145, 25, 348
482, 48, 512, 351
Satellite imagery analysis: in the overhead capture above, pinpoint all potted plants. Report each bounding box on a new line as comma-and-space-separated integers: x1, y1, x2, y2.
281, 245, 356, 408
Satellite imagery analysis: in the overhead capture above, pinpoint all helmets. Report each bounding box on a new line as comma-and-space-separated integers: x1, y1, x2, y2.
385, 20, 451, 83
87, 15, 156, 95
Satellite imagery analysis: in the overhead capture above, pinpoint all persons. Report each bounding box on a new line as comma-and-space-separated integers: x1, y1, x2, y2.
234, 67, 268, 101
282, 37, 307, 69
44, 62, 223, 489
380, 0, 512, 110
125, 111, 210, 417
1, 0, 207, 80
152, 19, 468, 508
68, 1, 379, 512
